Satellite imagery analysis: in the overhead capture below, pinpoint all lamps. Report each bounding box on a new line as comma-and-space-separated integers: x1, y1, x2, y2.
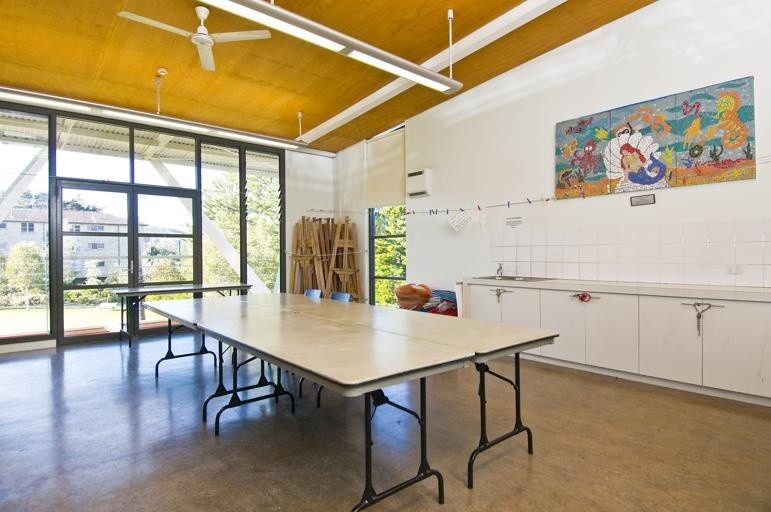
197, 0, 464, 97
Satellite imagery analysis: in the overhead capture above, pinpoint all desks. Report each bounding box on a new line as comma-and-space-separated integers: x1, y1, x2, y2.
228, 295, 561, 487
143, 296, 475, 512
110, 283, 253, 347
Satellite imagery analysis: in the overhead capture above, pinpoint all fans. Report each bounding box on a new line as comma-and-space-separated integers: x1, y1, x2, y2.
116, 5, 272, 73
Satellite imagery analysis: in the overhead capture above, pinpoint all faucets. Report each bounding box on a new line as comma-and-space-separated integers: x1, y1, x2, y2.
497, 262, 503, 276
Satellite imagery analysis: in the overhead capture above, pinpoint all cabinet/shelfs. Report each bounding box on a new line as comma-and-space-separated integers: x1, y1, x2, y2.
461, 276, 771, 407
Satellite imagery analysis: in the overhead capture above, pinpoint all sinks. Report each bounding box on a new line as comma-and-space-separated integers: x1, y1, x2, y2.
472, 275, 559, 282
